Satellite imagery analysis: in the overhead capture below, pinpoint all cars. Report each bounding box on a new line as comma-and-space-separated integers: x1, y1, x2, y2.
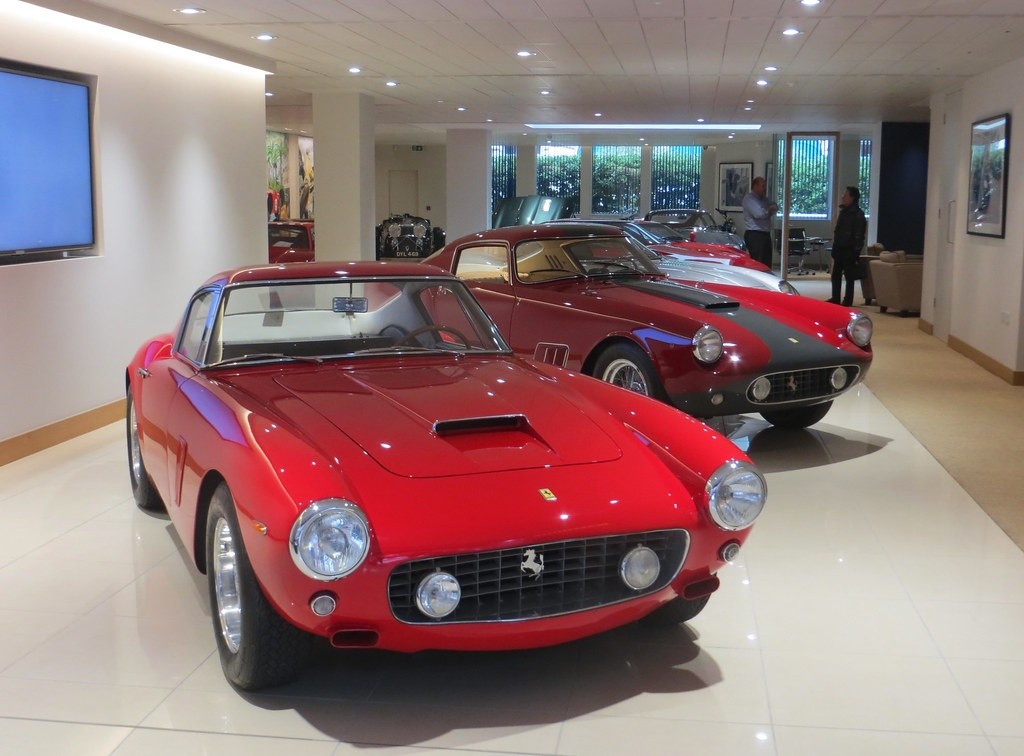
126, 260, 767, 691
268, 220, 317, 267
363, 223, 872, 430
569, 207, 800, 298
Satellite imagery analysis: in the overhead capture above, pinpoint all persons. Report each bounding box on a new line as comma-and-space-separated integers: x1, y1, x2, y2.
826, 186, 867, 307
743, 177, 778, 269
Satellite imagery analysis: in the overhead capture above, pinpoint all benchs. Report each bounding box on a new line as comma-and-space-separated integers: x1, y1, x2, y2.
193, 325, 422, 372
456, 272, 561, 284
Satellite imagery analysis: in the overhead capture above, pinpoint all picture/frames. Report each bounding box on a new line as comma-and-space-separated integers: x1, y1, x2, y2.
965, 113, 1011, 239
765, 162, 774, 203
718, 162, 754, 212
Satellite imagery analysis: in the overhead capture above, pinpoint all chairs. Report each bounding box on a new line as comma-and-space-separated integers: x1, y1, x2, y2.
772, 227, 817, 276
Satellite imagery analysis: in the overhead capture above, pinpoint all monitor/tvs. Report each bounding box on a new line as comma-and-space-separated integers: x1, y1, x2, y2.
0, 58, 102, 267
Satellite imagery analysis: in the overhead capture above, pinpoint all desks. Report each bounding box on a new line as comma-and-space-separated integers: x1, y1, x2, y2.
805, 235, 832, 273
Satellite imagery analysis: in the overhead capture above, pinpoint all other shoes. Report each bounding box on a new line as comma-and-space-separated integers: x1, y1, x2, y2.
840, 298, 853, 307
826, 295, 840, 304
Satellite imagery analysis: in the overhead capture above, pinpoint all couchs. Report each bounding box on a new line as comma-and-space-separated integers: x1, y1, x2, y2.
859, 242, 923, 318
